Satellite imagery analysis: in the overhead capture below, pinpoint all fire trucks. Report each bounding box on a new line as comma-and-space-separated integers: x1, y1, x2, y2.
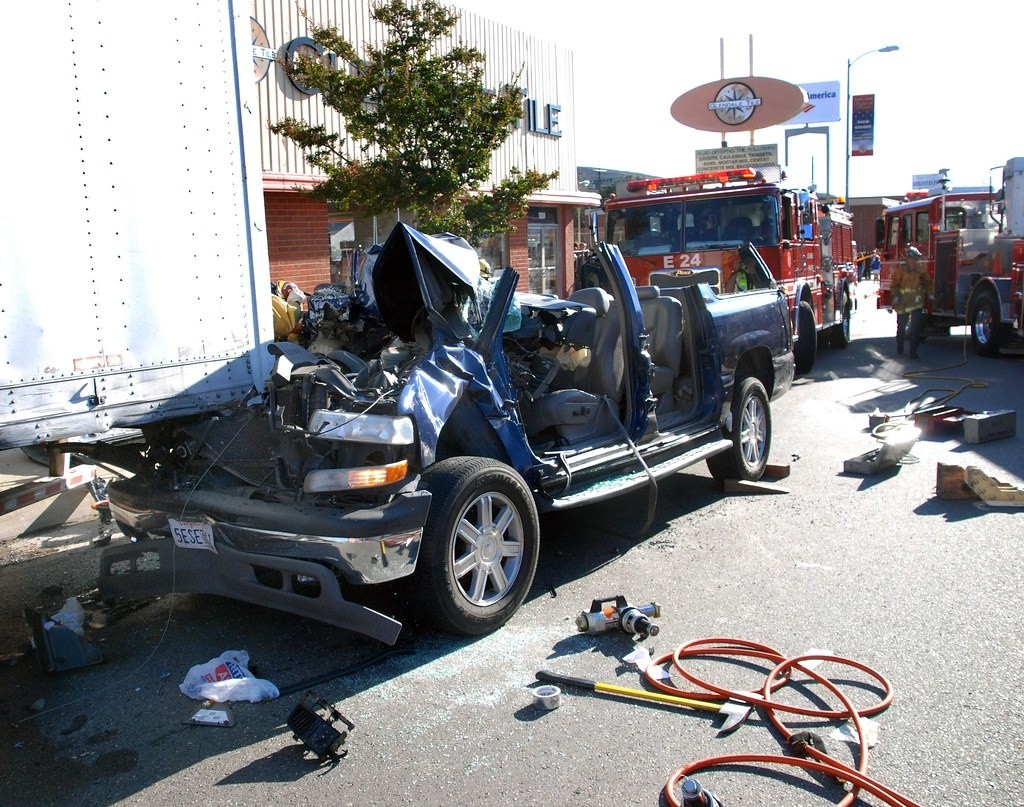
590, 165, 859, 376
873, 189, 1024, 358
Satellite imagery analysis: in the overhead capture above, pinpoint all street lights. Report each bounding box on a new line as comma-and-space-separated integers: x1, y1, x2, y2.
843, 44, 900, 212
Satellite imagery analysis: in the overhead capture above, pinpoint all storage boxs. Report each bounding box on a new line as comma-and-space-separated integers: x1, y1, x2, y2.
914, 406, 980, 444
843, 425, 922, 472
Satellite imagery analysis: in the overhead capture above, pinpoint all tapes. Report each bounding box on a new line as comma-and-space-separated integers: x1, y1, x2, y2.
532, 685, 561, 710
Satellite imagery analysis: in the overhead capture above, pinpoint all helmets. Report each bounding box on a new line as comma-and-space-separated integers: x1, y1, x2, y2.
904, 246, 922, 257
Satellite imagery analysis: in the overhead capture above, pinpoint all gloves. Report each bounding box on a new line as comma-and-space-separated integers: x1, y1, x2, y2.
287, 289, 305, 305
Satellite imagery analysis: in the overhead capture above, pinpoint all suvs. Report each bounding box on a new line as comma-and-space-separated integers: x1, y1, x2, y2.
87, 218, 802, 648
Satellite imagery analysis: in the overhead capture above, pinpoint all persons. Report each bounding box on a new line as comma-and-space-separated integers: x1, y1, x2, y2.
872, 255, 882, 274
890, 246, 936, 359
272, 289, 304, 343
578, 243, 588, 262
574, 243, 581, 291
864, 250, 872, 280
697, 214, 719, 241
857, 252, 865, 283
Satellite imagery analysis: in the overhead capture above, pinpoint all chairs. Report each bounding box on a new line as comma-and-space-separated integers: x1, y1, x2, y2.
522, 286, 681, 446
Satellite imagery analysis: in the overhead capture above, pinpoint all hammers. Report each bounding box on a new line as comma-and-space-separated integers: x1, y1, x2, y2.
535, 670, 765, 734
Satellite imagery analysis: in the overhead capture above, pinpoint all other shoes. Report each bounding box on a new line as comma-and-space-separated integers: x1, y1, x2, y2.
897, 342, 904, 353
911, 348, 919, 360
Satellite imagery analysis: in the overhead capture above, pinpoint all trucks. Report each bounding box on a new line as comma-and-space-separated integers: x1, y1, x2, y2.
0, 0, 283, 456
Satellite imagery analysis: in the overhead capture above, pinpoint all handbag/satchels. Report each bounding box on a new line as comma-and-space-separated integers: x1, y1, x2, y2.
198, 677, 280, 703
179, 650, 255, 701
